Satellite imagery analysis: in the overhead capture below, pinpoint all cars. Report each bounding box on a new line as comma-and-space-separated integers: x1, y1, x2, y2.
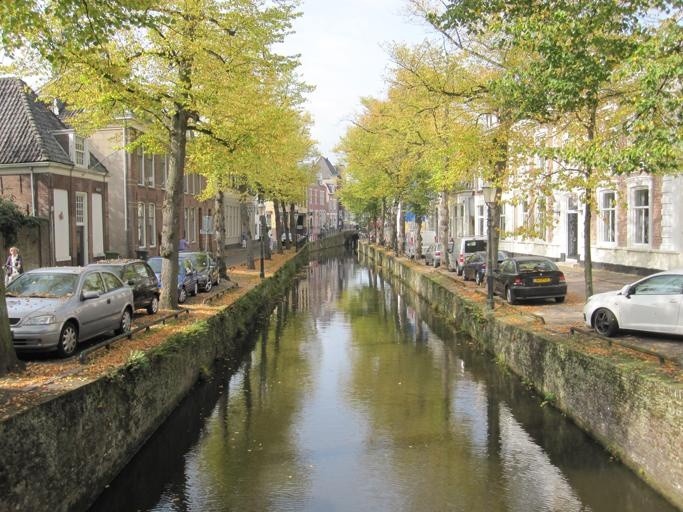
368, 226, 567, 305
3, 252, 220, 360
583, 268, 682, 340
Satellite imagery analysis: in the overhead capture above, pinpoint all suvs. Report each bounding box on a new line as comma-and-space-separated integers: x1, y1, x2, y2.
281, 233, 292, 245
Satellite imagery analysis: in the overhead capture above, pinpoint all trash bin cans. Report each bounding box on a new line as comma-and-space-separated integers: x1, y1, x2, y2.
135, 250, 148, 259
105, 251, 120, 259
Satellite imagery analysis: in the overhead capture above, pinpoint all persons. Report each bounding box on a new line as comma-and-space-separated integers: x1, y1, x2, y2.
240, 231, 248, 249
449, 237, 455, 244
319, 225, 326, 240
179, 236, 189, 253
2, 246, 23, 287
337, 216, 343, 231
